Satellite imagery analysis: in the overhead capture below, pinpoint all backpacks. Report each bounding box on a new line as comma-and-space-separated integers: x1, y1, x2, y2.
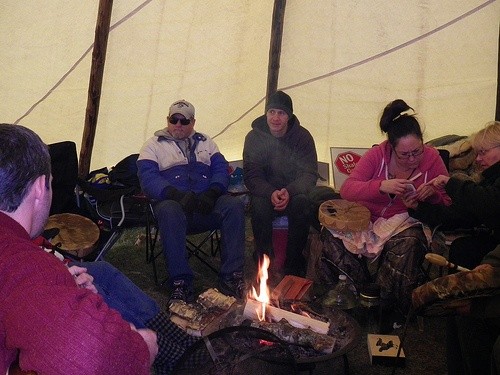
82, 155, 139, 208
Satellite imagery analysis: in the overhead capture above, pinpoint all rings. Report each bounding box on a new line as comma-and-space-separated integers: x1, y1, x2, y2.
435, 179, 440, 182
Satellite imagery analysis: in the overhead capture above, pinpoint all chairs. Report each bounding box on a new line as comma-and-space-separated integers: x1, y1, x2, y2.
108, 153, 224, 292
46, 140, 104, 263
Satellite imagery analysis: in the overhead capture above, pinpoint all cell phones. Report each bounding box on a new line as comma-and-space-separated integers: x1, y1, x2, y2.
406, 184, 416, 196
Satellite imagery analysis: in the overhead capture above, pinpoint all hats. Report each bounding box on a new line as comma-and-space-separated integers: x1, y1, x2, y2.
169, 99, 195, 119
265, 90, 293, 117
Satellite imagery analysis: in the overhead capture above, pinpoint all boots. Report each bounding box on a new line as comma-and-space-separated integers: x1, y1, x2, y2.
142, 311, 212, 374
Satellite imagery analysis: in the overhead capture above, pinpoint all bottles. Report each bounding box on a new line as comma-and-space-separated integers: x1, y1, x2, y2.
356, 273, 381, 329
332, 274, 357, 318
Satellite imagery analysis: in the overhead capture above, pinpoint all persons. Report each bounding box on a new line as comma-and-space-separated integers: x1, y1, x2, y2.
136, 99, 244, 301
404, 120, 500, 276
243, 91, 318, 274
0, 123, 160, 375
321, 99, 453, 299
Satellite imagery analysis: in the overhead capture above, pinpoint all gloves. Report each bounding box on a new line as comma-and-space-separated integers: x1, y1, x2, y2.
174, 187, 220, 216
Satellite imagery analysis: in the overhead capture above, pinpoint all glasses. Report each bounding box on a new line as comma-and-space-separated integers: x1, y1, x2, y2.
169, 116, 190, 125
472, 144, 500, 159
393, 139, 424, 160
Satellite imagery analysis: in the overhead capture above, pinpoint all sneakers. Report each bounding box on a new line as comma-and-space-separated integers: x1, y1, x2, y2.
167, 278, 195, 306
221, 271, 247, 300
